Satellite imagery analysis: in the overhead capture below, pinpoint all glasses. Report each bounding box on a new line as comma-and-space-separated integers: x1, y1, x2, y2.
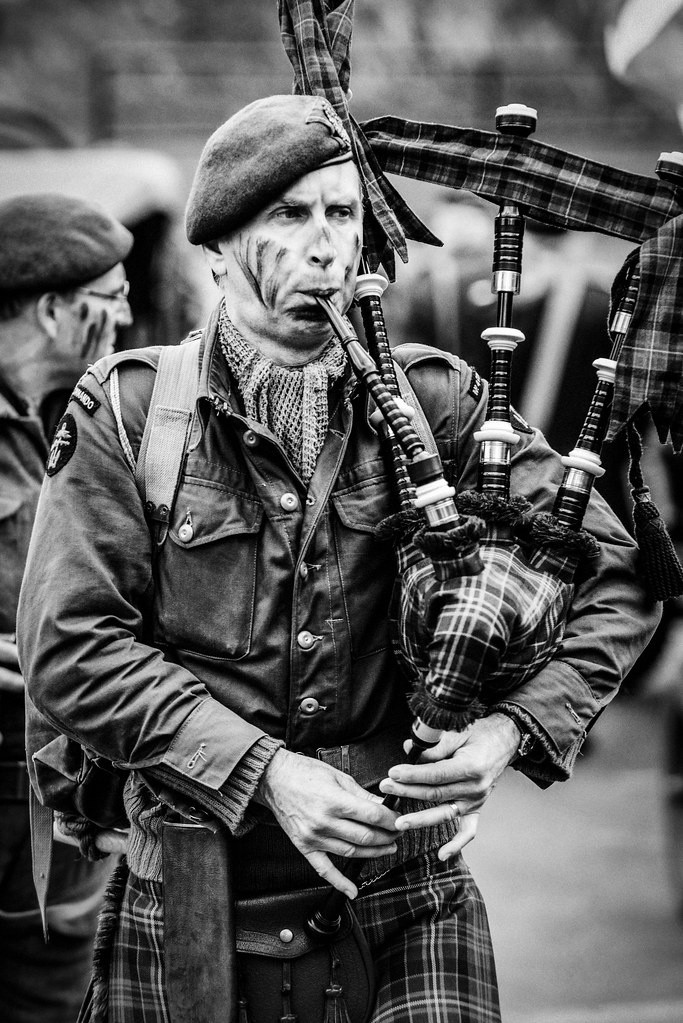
76, 281, 130, 302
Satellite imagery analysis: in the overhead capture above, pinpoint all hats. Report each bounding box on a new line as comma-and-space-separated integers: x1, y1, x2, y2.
0, 194, 134, 318
183, 93, 351, 246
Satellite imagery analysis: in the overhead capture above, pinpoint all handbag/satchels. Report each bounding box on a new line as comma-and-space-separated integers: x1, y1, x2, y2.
228, 882, 375, 1022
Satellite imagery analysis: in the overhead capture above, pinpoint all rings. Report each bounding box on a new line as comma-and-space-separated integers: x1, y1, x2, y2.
449, 802, 459, 815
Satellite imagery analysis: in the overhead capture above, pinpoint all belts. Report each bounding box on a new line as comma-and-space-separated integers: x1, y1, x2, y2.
304, 727, 412, 789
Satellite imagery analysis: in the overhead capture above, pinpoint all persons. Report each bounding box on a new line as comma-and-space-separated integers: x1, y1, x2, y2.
0, 95, 664, 1023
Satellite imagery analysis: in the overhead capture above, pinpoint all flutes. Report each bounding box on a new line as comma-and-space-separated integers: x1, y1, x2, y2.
303, 99, 682, 946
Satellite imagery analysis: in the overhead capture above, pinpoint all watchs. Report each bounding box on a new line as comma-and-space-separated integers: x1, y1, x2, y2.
507, 714, 536, 756
